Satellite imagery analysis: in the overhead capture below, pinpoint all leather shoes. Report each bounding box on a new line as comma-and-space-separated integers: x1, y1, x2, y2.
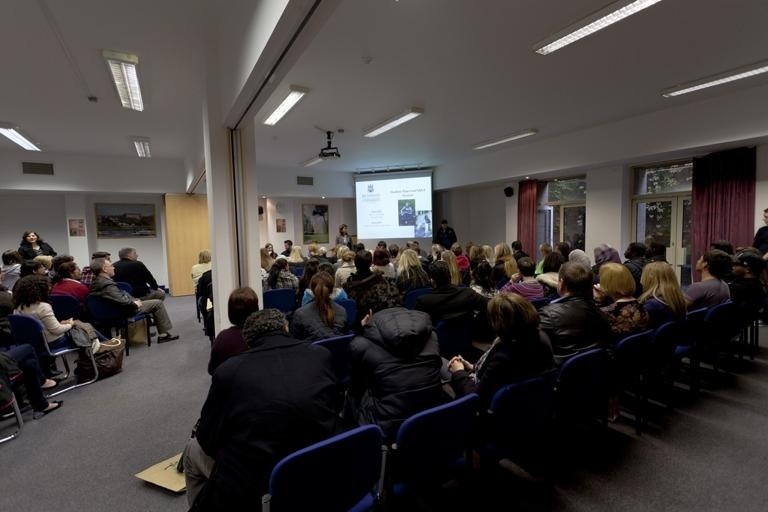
32, 399, 63, 420
41, 377, 63, 390
151, 332, 179, 343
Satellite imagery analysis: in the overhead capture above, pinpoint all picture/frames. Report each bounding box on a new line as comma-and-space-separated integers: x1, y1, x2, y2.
302, 203, 329, 245
93, 202, 158, 238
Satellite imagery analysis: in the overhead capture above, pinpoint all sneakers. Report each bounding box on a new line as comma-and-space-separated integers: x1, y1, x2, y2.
2, 402, 29, 418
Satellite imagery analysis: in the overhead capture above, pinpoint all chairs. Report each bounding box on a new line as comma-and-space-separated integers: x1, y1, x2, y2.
0, 282, 150, 445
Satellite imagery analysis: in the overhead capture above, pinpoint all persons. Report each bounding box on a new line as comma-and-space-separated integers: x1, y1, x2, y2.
0, 230, 179, 421
182, 239, 768, 512
433, 219, 457, 250
751, 208, 768, 254
335, 224, 352, 246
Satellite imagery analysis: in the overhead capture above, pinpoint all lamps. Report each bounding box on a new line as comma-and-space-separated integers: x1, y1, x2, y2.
303, 155, 322, 168
661, 59, 768, 100
102, 48, 144, 111
364, 107, 425, 138
263, 85, 310, 128
0, 122, 42, 152
534, 1, 660, 56
134, 137, 152, 160
472, 128, 539, 151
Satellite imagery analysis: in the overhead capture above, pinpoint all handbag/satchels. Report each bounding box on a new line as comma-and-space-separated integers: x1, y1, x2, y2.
73, 334, 129, 381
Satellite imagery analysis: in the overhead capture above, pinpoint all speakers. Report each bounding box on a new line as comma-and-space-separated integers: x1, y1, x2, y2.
258, 206, 264, 215
504, 186, 514, 198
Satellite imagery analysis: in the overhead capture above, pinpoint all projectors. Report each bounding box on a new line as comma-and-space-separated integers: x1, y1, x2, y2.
319, 151, 340, 161
